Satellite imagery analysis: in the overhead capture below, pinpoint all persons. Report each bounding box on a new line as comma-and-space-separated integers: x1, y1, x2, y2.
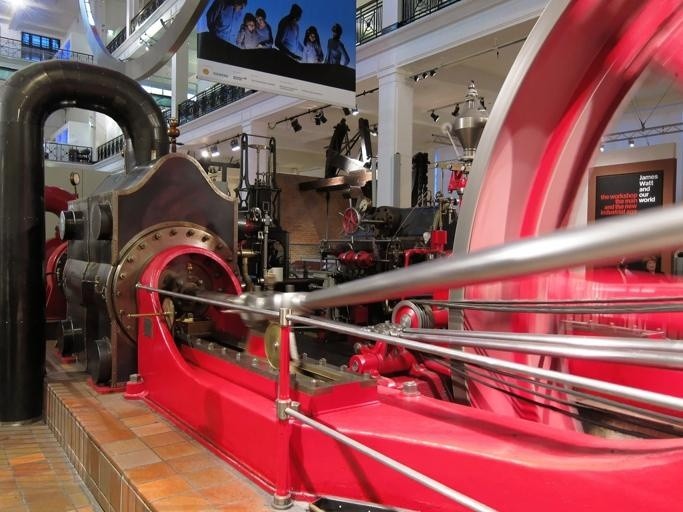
235, 8, 274, 50
206, 0, 248, 46
325, 23, 350, 66
275, 5, 303, 59
642, 255, 665, 275
433, 197, 459, 230
301, 26, 324, 64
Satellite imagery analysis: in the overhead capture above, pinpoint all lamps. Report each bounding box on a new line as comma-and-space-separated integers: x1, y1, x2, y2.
412, 69, 436, 83
208, 137, 239, 158
429, 97, 485, 122
289, 100, 358, 134
